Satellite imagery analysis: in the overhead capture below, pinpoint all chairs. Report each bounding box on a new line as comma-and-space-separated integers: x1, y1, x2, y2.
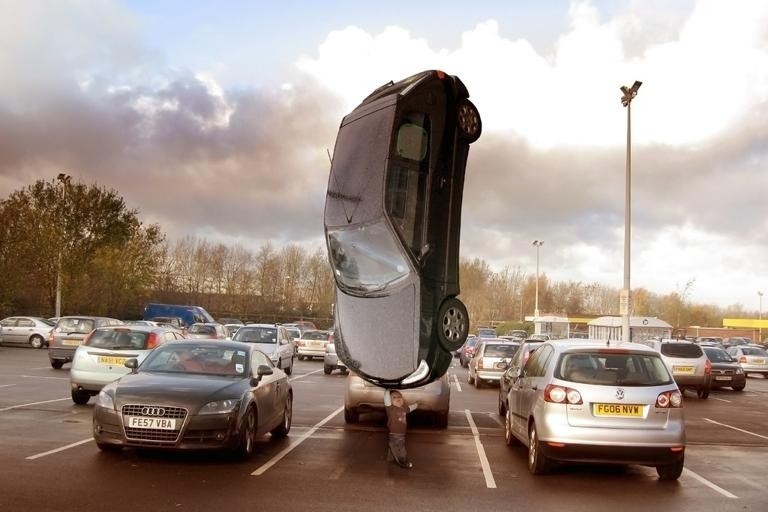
223, 351, 244, 375
118, 335, 130, 346
571, 364, 648, 383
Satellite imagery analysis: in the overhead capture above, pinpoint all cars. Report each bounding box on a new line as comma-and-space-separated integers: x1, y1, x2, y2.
92, 340, 294, 462
318, 67, 484, 390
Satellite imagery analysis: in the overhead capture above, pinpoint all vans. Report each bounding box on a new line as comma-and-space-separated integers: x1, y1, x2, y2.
502, 336, 686, 484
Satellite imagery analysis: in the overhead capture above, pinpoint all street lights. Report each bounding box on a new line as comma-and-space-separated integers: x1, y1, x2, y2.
756, 289, 763, 343
280, 275, 292, 309
618, 80, 642, 339
531, 238, 544, 330
56, 172, 73, 318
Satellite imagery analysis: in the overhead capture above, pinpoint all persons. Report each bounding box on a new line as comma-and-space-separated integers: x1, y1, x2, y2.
177, 328, 192, 340
383, 389, 422, 469
